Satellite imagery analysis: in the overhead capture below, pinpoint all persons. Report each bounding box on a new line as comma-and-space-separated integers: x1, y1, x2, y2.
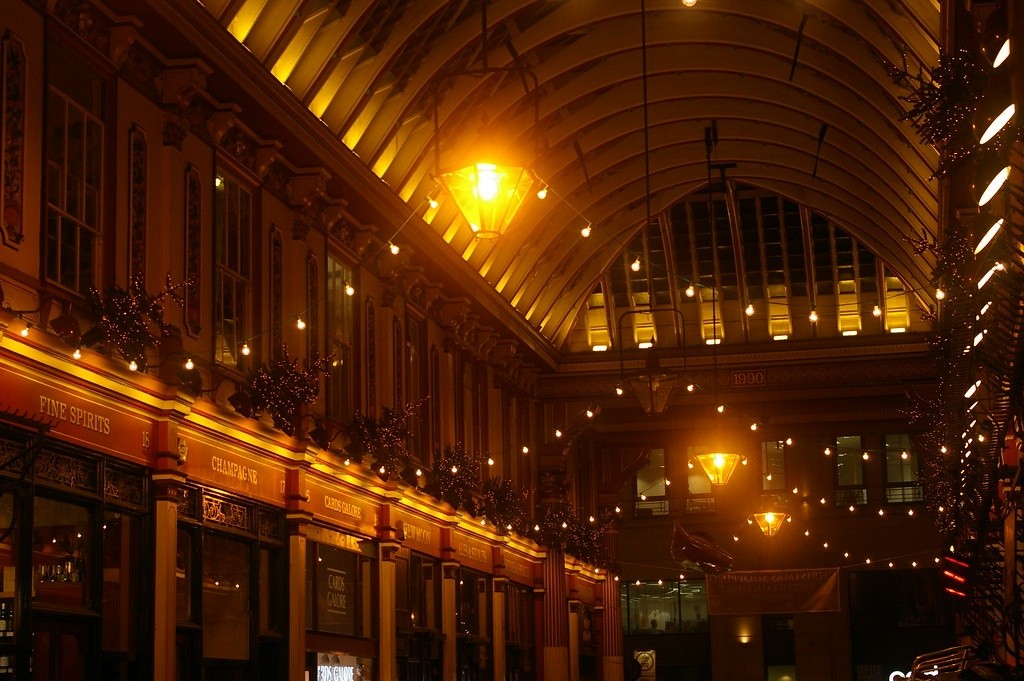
649, 620, 661, 633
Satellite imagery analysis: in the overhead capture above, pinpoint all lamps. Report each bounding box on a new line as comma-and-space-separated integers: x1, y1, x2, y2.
971, 88, 1015, 145
364, 451, 390, 482
970, 148, 1010, 208
754, 404, 788, 537
960, 255, 1015, 451
202, 376, 252, 418
620, 1, 689, 416
12, 295, 81, 349
691, 124, 748, 487
967, 206, 1003, 255
401, 467, 544, 546
279, 413, 296, 438
299, 414, 329, 451
983, 13, 1010, 69
329, 429, 363, 464
146, 351, 202, 395
431, 0, 549, 238
